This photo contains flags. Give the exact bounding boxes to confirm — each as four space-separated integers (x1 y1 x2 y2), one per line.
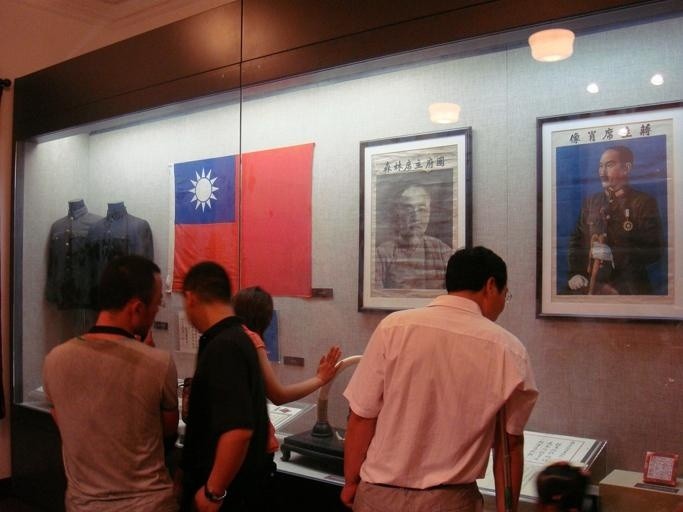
165 140 314 303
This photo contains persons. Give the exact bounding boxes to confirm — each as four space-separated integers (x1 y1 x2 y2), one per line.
233 284 342 511
173 261 272 511
42 254 181 510
42 200 154 311
534 462 592 512
340 246 540 511
375 184 452 288
565 145 664 294
132 260 163 348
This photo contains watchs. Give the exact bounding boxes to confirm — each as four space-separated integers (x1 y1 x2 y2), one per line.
203 483 228 503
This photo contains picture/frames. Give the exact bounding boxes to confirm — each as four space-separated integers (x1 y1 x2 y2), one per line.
358 125 473 313
536 108 682 323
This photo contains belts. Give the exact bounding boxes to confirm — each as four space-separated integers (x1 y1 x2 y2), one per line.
376 481 477 492
